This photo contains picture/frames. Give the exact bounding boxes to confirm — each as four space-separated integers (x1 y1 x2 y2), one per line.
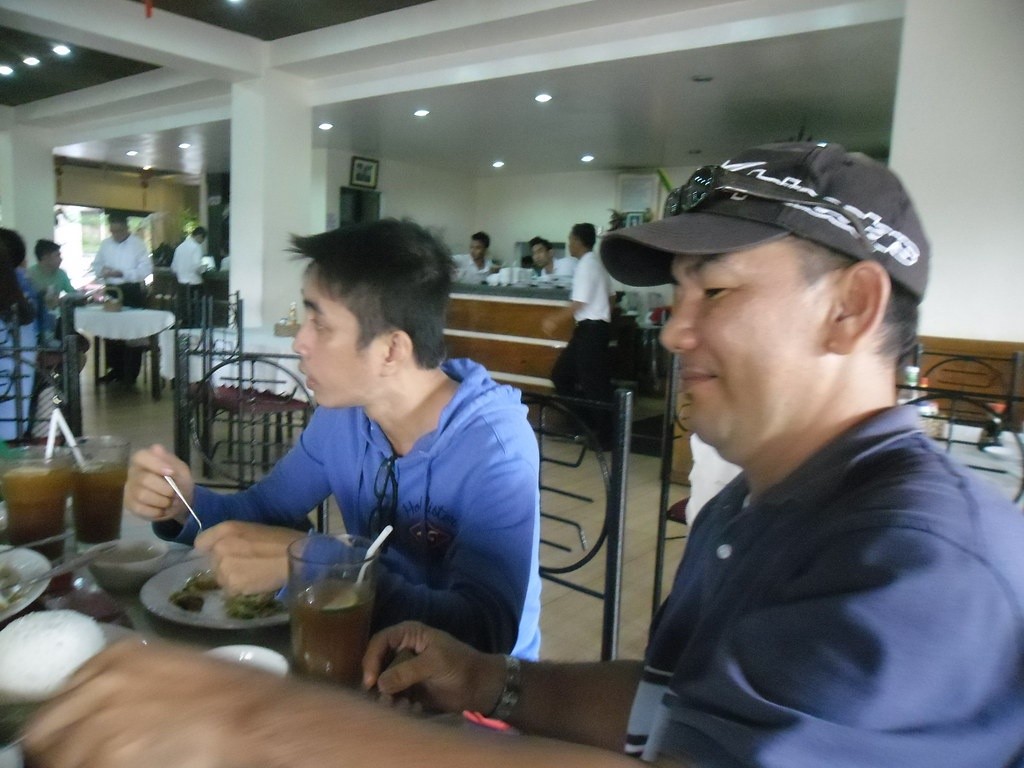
625 212 643 227
348 156 380 189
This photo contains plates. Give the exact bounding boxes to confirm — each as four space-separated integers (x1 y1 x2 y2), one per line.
71 542 190 596
136 553 289 630
0 543 52 622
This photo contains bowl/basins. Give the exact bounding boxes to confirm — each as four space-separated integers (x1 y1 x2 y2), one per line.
201 644 291 679
487 266 535 287
82 538 168 592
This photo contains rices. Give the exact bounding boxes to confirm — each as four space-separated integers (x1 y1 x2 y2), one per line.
0 608 106 708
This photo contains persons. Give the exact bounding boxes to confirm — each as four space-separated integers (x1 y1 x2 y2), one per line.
527 236 578 277
121 216 541 662
170 226 208 328
446 231 493 283
0 227 91 375
19 140 1024 768
92 212 154 384
549 223 617 429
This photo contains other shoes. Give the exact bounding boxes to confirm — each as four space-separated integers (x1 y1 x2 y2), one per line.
98 370 137 386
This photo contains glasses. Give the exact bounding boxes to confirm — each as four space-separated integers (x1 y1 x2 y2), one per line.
367 454 406 553
662 164 872 255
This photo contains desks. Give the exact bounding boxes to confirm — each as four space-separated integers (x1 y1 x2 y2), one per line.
686 422 1024 526
74 302 176 402
160 327 313 408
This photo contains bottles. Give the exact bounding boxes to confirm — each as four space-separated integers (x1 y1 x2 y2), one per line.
977 403 1004 450
893 366 920 407
288 302 297 329
918 377 929 400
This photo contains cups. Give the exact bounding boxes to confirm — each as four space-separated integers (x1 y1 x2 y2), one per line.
63 434 131 542
287 533 381 688
0 445 73 569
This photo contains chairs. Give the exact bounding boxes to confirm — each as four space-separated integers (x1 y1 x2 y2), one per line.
0 284 1024 663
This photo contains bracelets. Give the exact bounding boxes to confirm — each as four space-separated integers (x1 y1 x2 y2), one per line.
488 653 522 720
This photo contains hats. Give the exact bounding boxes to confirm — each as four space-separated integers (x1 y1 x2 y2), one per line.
599 141 932 302
